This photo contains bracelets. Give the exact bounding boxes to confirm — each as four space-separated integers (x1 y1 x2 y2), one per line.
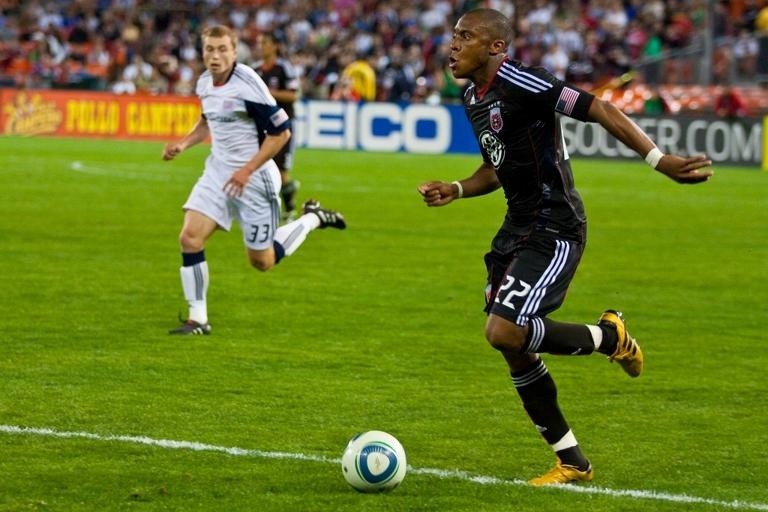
451 177 465 199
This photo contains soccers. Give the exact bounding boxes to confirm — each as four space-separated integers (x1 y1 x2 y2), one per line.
341 428 406 492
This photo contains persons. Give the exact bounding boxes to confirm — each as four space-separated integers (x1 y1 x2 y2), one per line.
162 25 347 335
0 0 768 116
416 9 715 487
247 29 301 225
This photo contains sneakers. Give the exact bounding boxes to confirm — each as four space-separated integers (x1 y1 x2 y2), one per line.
304 200 345 229
597 309 643 377
529 457 592 486
285 209 296 223
171 320 210 335
